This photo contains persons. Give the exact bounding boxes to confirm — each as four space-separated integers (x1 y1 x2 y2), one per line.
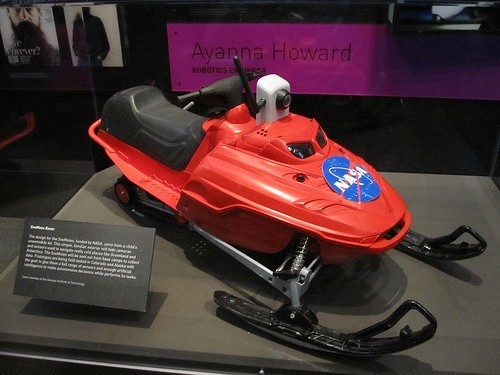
6 4 61 66
72 5 110 67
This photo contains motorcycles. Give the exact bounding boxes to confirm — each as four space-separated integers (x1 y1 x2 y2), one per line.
88 70 489 362
